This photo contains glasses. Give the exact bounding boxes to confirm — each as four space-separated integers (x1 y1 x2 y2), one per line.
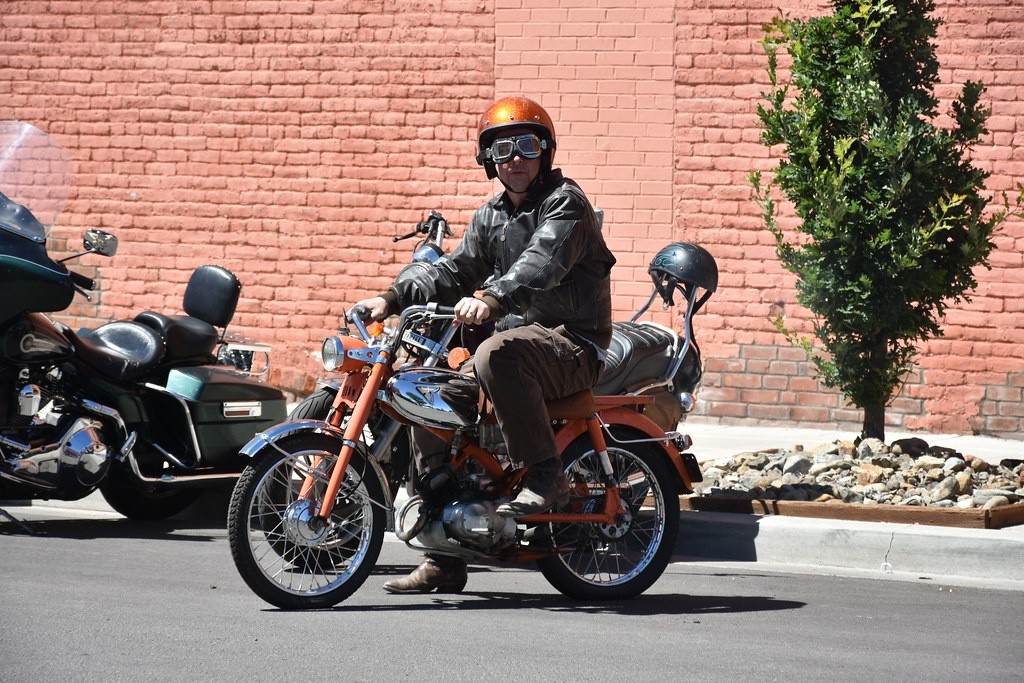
476 133 557 165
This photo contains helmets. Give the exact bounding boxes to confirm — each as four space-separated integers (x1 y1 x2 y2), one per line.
476 96 556 180
648 242 718 293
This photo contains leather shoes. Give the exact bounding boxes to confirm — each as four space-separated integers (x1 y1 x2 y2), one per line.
495 455 571 516
382 556 468 593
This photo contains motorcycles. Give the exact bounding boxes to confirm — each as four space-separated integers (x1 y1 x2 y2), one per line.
230 212 719 614
0 120 287 537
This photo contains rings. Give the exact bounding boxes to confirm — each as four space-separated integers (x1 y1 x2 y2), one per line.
468 312 475 315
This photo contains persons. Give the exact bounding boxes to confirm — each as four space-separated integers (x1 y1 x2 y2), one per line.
347 95 617 595
